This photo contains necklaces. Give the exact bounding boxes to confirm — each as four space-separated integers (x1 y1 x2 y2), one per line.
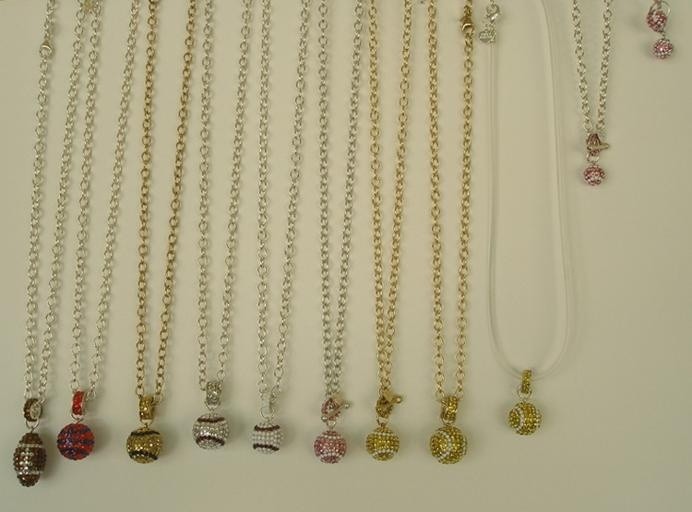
126 1 196 463
314 0 363 462
427 0 474 462
57 2 137 460
572 0 612 184
488 0 569 435
367 1 413 462
250 1 310 453
13 3 86 488
192 0 253 449
